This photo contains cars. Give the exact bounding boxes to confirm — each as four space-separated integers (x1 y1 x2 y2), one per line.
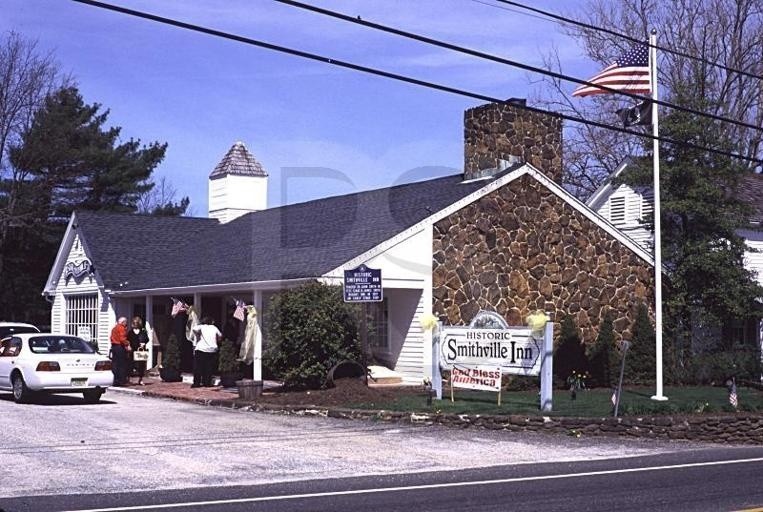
0 332 116 403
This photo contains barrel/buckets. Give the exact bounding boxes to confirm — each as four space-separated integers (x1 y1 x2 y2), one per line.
235 380 263 400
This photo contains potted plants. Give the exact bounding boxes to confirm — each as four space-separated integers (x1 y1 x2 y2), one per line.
159 332 183 382
217 338 240 387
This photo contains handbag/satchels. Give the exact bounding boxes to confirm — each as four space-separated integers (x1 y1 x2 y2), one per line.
133 347 150 362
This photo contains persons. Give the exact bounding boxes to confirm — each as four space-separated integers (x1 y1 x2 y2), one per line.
189 317 223 388
110 316 150 387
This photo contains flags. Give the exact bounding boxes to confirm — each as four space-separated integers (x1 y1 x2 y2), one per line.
170 296 189 319
232 300 246 322
728 382 738 409
570 40 652 98
610 389 617 407
